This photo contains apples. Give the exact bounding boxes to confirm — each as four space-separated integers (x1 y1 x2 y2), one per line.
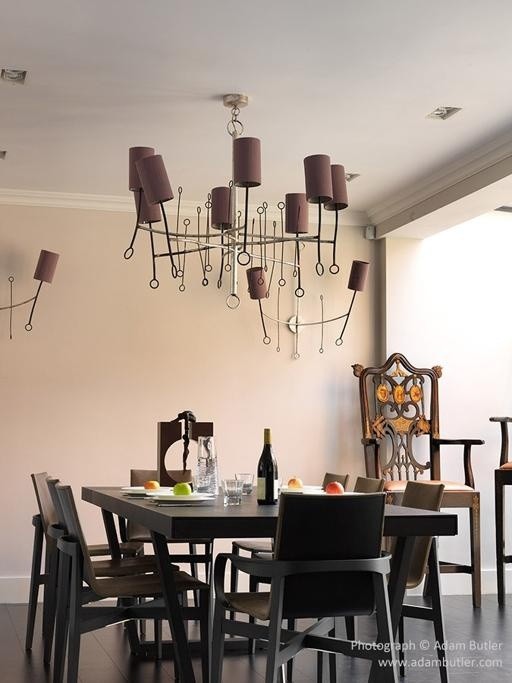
326 482 344 494
174 483 192 495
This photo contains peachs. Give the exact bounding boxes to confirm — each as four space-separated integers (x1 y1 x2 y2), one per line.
144 480 159 489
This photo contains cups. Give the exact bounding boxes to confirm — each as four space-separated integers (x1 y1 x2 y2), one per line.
235 474 254 496
192 475 212 493
197 436 220 497
221 479 244 507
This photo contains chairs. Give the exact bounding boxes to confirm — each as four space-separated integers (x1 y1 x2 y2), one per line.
487 410 512 616
22 466 459 682
352 350 487 619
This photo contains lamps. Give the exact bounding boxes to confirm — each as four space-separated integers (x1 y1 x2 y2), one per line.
115 89 354 310
242 253 371 357
1 246 60 343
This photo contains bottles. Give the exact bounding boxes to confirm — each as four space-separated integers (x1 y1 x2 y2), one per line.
256 427 279 506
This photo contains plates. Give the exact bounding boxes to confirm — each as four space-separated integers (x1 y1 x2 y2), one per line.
282 485 363 495
119 486 172 495
141 492 216 504
